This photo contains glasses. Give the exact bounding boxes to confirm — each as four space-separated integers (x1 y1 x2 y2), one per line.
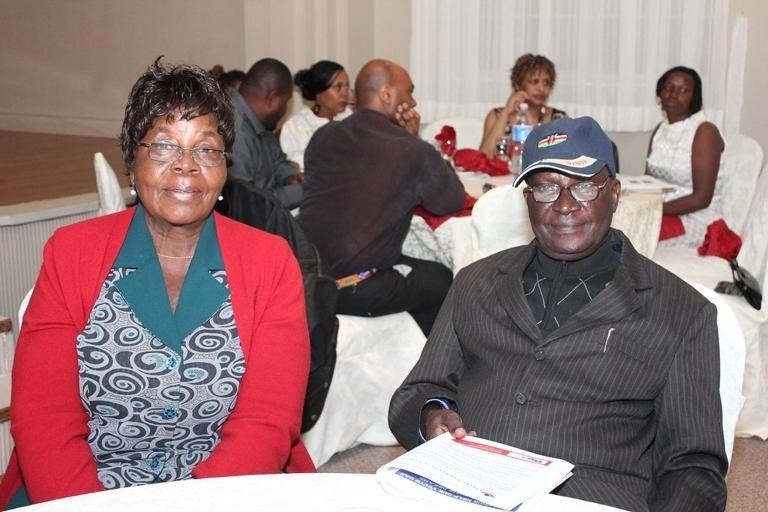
529 182 604 203
141 144 228 167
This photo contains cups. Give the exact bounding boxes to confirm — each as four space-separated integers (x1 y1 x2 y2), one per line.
439 139 457 162
495 136 510 156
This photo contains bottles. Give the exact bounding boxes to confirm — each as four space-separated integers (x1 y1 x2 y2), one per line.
511 104 531 176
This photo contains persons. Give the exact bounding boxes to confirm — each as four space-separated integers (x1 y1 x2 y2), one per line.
642 65 725 248
387 116 728 511
211 58 357 247
479 50 566 174
292 57 464 337
1 56 320 512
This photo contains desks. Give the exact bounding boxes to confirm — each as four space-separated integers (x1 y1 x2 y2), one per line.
444 159 748 483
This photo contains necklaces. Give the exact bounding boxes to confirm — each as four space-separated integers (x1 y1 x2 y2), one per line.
158 253 193 260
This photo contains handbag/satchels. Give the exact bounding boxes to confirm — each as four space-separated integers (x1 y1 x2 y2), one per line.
730 259 761 309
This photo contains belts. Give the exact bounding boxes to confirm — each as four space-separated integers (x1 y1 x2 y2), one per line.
334 268 379 290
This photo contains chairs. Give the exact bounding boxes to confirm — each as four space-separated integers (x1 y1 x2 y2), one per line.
665 127 766 441
92 149 439 474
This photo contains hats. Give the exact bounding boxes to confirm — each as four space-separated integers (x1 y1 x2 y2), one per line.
512 116 616 188
